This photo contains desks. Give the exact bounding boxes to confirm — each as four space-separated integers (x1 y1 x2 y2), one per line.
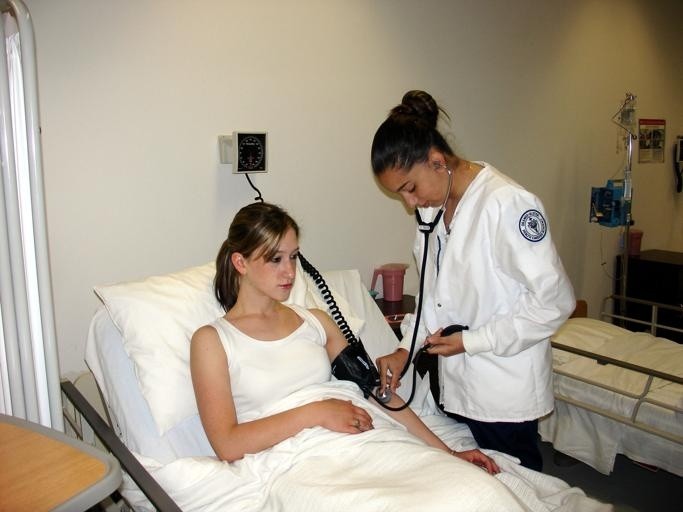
0 413 123 512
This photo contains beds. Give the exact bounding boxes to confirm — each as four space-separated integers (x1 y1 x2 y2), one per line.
537 294 683 478
59 269 612 512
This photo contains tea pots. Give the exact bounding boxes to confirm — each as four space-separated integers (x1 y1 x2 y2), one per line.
371 263 409 302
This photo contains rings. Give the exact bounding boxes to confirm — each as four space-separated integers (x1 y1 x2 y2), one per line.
354 418 360 427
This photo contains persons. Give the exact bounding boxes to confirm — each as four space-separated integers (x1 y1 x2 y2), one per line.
371 88 579 470
187 199 502 476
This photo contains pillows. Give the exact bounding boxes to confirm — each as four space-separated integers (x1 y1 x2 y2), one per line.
94 258 366 437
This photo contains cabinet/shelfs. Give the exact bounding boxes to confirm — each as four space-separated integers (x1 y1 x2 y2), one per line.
614 249 683 344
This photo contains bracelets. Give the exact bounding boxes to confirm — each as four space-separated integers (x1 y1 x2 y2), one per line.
450 450 457 456
